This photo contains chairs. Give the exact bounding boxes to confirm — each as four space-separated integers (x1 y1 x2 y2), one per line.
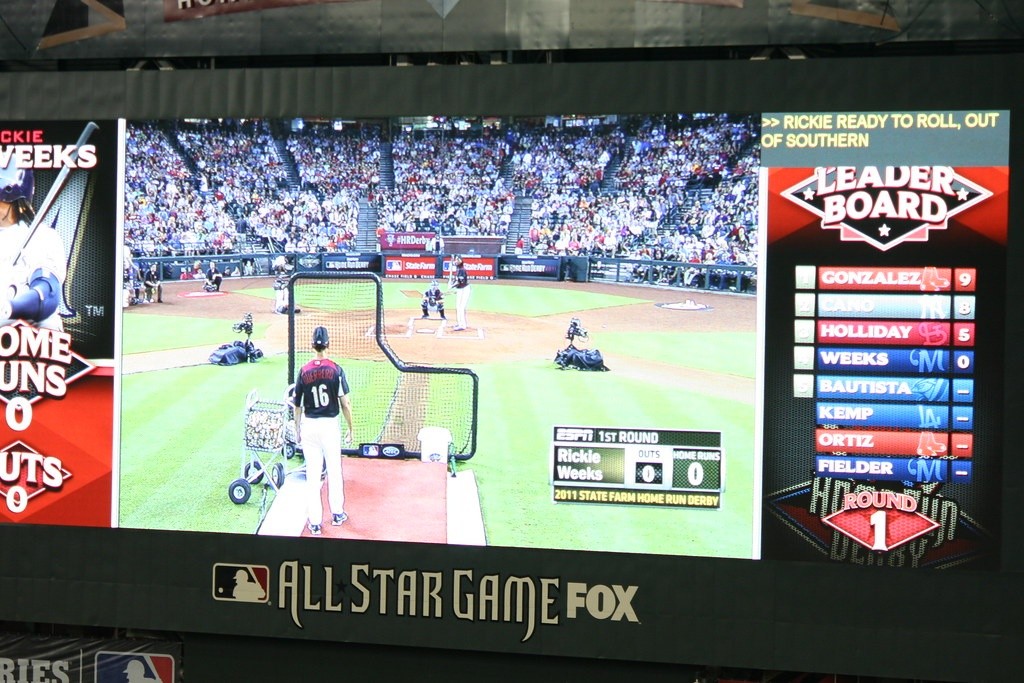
121 121 759 300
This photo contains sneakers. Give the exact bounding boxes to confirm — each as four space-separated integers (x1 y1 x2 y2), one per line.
332 513 347 526
308 523 321 535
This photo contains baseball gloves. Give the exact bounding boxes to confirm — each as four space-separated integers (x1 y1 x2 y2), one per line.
429 298 436 306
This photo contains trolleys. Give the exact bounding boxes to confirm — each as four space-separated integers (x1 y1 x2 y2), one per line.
227 386 291 504
279 383 305 460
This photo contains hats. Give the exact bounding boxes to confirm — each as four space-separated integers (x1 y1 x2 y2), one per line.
312 326 329 345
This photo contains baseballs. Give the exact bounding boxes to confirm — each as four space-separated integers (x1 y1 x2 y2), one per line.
246 411 286 448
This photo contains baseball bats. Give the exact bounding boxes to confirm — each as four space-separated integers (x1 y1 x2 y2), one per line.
10 122 98 269
448 253 455 290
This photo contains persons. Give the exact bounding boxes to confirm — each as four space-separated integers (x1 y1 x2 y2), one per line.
205 262 222 292
450 255 470 331
420 281 447 321
125 125 758 290
2 154 66 326
143 264 163 303
291 327 355 536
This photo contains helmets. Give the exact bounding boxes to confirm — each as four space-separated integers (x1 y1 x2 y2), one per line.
430 281 439 292
454 255 463 266
1 152 36 204
